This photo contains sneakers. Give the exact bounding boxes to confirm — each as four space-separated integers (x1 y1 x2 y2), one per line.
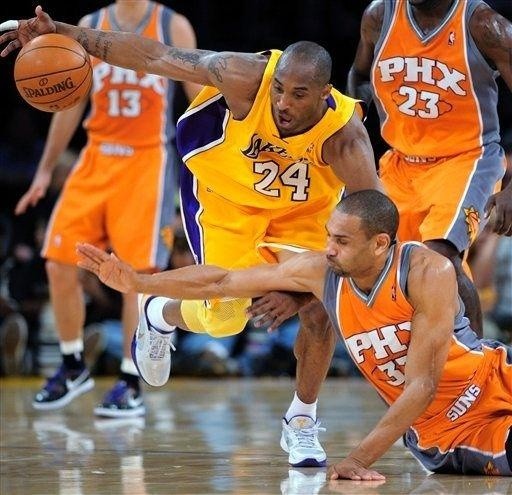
94 379 147 417
130 293 177 390
280 412 330 467
31 367 94 411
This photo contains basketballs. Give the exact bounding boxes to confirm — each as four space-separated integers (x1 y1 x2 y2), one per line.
14 33 94 112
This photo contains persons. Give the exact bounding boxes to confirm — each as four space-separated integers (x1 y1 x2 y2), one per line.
75 189 512 482
0 6 381 466
14 0 207 418
342 1 512 341
